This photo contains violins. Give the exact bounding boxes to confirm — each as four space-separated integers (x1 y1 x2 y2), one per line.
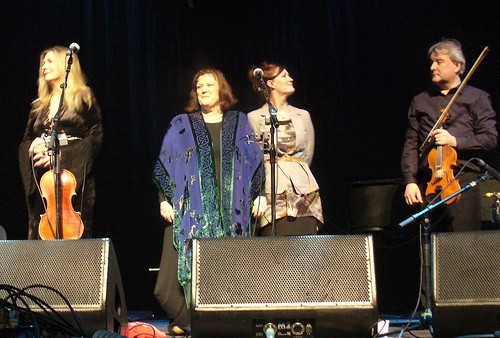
37 118 85 240
425 109 462 207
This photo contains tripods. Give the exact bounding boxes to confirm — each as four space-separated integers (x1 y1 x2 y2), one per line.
380 169 493 337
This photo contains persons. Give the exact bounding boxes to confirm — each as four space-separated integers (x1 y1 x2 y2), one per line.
17 46 102 240
247 64 324 237
401 41 499 311
153 67 267 336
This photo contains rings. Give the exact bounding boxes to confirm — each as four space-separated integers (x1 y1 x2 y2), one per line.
42 151 46 158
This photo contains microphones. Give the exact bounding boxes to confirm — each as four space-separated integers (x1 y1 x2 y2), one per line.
476 157 500 183
69 42 80 53
252 67 266 90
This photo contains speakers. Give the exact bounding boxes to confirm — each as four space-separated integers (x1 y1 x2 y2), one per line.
428 230 500 338
0 238 129 338
189 234 378 338
